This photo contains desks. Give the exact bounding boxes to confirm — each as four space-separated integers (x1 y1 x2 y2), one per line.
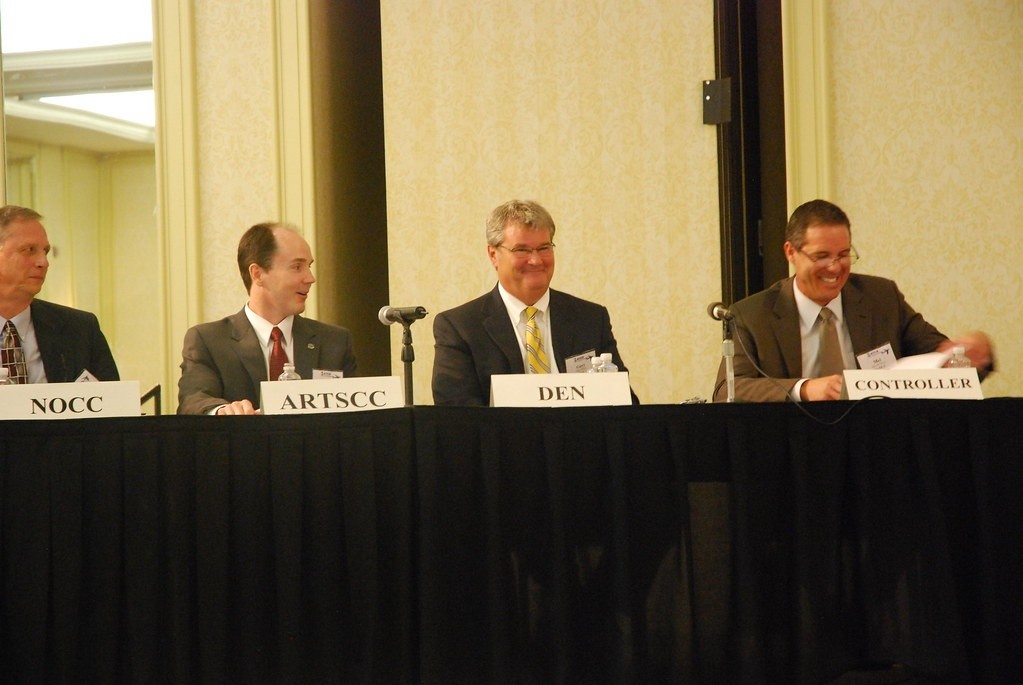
1 396 1022 685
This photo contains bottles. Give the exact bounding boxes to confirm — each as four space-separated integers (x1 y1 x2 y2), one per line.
599 351 618 372
584 356 608 372
277 362 301 380
947 346 971 368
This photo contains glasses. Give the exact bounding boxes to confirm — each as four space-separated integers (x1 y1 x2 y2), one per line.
799 245 858 267
499 242 555 257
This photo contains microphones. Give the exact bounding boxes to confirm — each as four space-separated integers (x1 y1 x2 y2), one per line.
707 301 734 322
378 305 429 327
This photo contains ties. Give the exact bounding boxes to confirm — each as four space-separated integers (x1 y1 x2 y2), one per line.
522 306 551 373
811 308 849 400
1 320 30 384
270 326 288 381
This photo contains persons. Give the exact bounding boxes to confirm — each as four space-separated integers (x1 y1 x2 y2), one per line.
711 200 992 402
176 222 363 415
0 205 120 384
431 200 640 407
